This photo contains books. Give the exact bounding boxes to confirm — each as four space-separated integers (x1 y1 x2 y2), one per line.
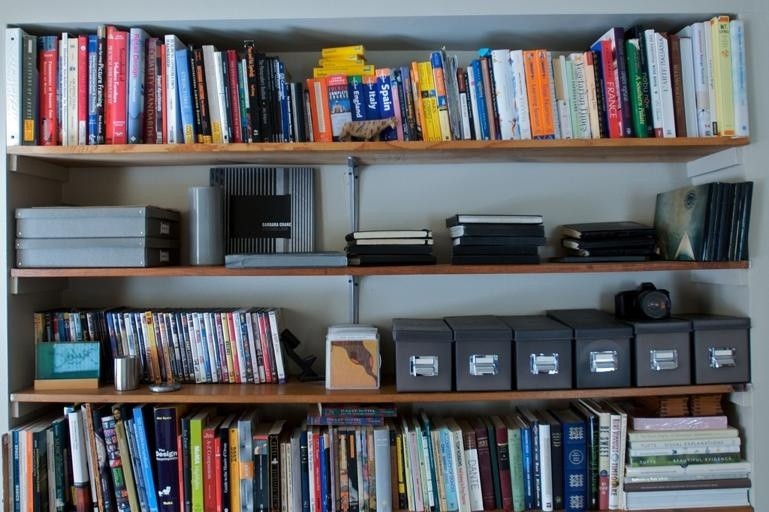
653 181 753 262
446 213 546 266
344 229 437 267
549 222 657 263
31 306 288 386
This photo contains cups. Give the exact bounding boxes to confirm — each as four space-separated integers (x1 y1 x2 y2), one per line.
113 354 140 392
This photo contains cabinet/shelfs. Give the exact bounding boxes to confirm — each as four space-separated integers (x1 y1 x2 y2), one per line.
0 1 769 511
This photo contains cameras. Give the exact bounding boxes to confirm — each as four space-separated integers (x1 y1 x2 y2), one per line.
614 283 671 323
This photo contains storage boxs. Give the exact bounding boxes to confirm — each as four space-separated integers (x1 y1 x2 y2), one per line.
675 311 750 385
392 318 453 393
326 327 382 390
444 315 513 392
616 311 692 388
547 308 631 389
15 239 180 268
501 314 575 390
14 206 181 238
